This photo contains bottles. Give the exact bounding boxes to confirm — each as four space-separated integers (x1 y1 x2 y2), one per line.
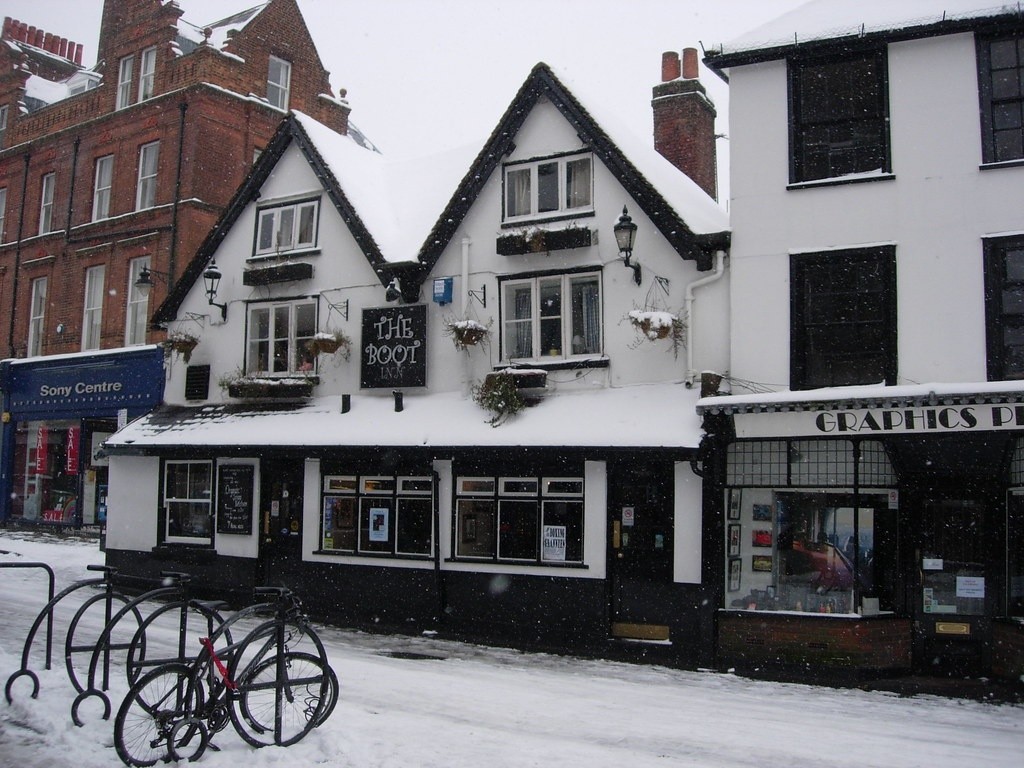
796 602 802 611
826 604 830 613
820 603 824 612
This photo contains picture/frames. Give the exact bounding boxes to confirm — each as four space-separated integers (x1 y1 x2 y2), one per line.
727 487 742 520
727 557 741 593
727 523 741 557
752 555 772 572
462 514 477 544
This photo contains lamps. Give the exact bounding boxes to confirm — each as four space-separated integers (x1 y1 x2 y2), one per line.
202 257 228 322
135 263 172 297
613 203 642 288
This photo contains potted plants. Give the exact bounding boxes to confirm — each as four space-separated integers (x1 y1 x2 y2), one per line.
436 297 495 360
305 323 354 365
219 365 314 398
162 332 199 366
617 279 691 361
471 372 546 429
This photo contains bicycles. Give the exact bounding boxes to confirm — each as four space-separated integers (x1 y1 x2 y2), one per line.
113 587 340 767
